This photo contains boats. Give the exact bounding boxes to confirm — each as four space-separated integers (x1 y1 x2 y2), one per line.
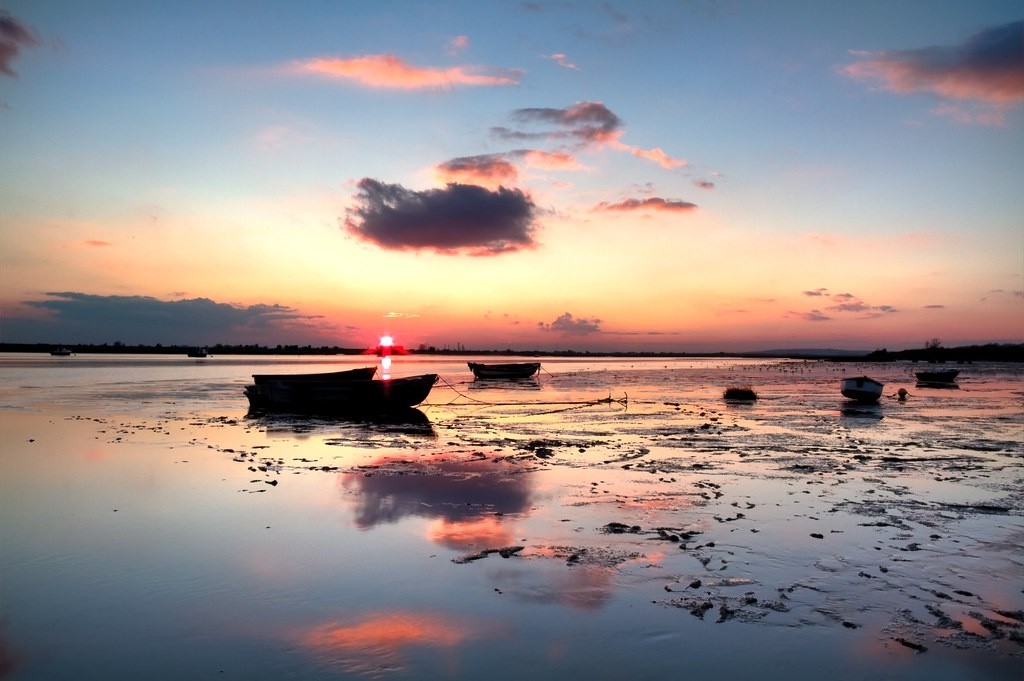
465 360 541 380
839 375 885 401
251 366 378 389
914 368 963 382
242 373 438 415
